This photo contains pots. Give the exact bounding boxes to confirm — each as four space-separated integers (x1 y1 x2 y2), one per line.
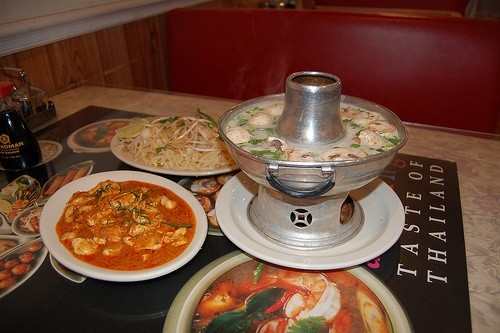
216 71 410 250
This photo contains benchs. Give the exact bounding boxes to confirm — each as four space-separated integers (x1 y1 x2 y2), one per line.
167 0 500 135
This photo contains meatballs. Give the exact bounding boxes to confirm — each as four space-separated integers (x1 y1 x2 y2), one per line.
0 242 41 290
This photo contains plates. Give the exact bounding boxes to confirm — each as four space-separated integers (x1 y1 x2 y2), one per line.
215 172 404 270
110 116 241 177
38 170 209 282
0 160 93 298
177 170 240 236
162 248 414 333
0 140 63 171
67 118 131 153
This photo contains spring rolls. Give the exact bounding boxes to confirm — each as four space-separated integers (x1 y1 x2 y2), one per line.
44 167 90 194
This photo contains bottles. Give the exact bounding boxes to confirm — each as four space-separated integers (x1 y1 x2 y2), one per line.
0 82 49 188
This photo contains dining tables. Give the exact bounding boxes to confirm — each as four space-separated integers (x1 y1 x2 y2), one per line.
0 80 500 333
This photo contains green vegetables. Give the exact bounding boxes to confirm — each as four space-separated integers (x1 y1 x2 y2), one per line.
197 264 327 333
0 177 41 209
156 109 224 151
95 183 193 227
